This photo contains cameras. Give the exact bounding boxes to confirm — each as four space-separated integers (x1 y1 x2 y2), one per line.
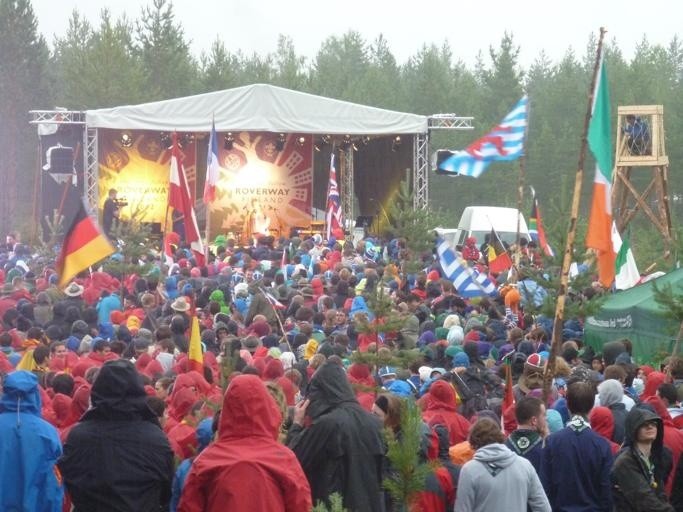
113 197 128 208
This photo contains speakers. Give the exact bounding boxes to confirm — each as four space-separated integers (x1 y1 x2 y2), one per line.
48 148 73 175
431 151 457 175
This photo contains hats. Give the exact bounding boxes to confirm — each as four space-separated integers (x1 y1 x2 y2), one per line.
379 365 397 376
65 282 84 297
277 285 290 299
525 355 547 370
171 297 190 311
302 289 315 297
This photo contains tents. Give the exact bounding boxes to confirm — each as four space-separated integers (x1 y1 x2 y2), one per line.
29 83 475 244
581 267 683 370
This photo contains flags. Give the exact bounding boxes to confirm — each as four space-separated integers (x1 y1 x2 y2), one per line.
326 151 346 241
480 226 532 292
187 296 205 369
170 135 206 268
435 90 530 181
257 285 290 311
540 26 641 290
54 194 121 290
527 188 558 260
202 123 223 252
434 231 507 318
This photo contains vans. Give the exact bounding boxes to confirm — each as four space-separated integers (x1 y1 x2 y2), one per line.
454 205 532 259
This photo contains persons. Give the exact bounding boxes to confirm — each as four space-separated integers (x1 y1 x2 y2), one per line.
506 396 547 469
1 233 51 391
471 274 510 444
644 397 683 502
142 235 222 465
423 381 471 449
291 355 387 510
51 284 180 430
399 412 461 511
179 375 312 511
612 406 671 512
63 360 174 511
539 382 615 512
456 419 551 512
498 288 683 444
375 231 484 413
2 370 63 510
206 231 379 415
102 187 118 234
370 393 405 436
622 116 651 158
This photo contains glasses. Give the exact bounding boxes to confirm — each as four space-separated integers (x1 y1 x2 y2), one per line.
641 420 659 427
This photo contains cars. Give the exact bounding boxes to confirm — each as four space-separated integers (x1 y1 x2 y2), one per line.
529 230 555 257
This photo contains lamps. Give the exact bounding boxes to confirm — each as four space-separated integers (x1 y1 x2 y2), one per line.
274 133 286 150
177 132 195 149
352 136 370 152
391 135 401 153
158 131 174 152
224 132 234 150
120 131 133 148
338 135 351 153
296 134 305 147
314 134 330 153
